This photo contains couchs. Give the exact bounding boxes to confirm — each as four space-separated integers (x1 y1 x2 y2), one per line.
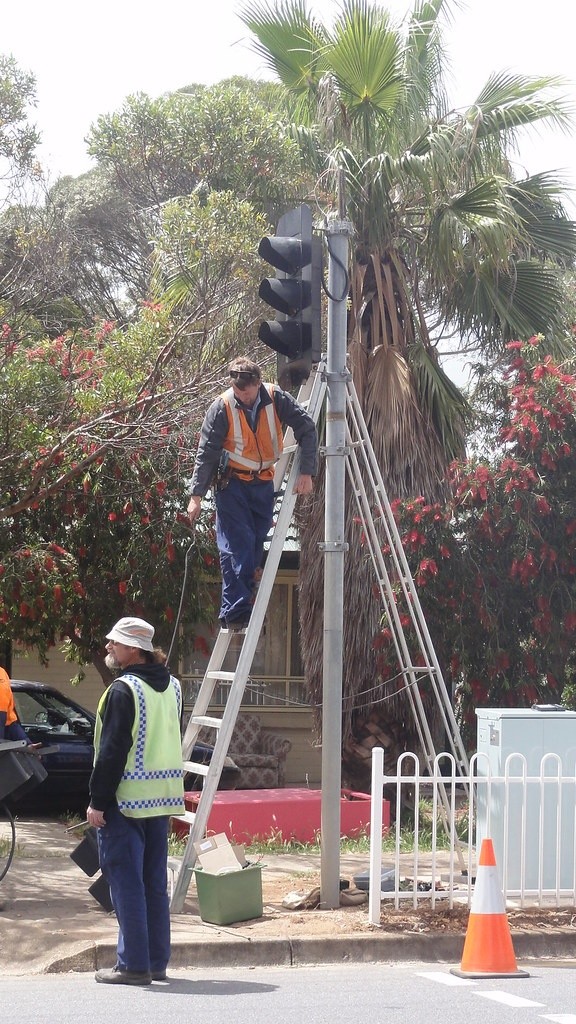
190 714 293 788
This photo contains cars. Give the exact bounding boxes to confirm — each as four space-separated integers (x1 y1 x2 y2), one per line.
10 678 242 812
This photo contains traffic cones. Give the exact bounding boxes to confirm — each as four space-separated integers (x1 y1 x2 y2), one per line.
449 839 531 979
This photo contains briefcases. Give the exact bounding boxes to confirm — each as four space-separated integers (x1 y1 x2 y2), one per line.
353 867 401 891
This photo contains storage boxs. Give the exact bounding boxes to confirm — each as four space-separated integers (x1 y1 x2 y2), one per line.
354 868 395 892
188 860 267 925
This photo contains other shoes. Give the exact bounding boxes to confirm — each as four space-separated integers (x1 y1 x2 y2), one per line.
220 611 268 629
96 964 151 985
151 969 167 979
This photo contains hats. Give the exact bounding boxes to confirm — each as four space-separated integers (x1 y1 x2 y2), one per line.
106 615 155 652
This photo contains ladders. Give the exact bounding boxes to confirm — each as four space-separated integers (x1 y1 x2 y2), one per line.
169 356 475 917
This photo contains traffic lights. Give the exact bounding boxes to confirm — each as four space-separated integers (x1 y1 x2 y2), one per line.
255 205 323 381
0 740 49 806
69 825 114 913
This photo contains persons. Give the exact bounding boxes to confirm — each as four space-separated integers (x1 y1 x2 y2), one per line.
84 614 188 987
1 664 43 762
186 354 320 631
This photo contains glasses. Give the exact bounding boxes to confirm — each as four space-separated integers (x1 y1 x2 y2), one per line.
110 639 120 646
230 370 260 380
